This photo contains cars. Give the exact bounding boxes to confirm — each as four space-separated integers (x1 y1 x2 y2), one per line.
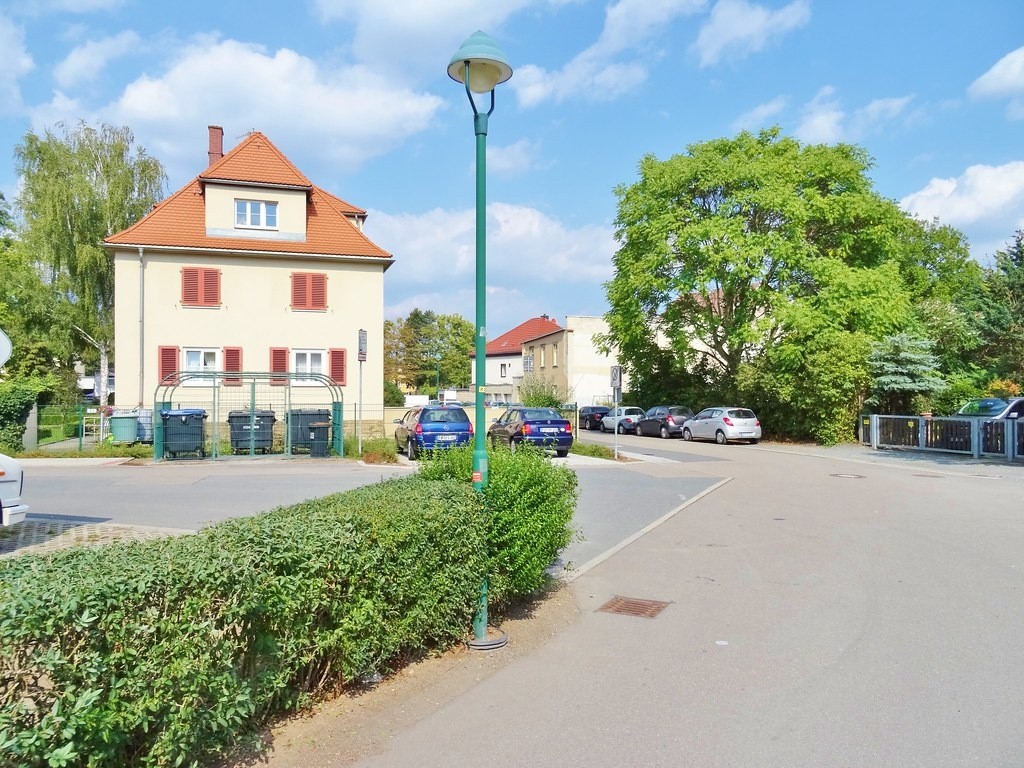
431 399 576 410
634 404 696 440
392 404 475 461
681 406 762 445
939 396 1024 450
599 406 646 434
578 405 612 431
486 405 574 457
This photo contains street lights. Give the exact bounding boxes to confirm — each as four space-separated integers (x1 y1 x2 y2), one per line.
434 353 442 405
445 28 515 640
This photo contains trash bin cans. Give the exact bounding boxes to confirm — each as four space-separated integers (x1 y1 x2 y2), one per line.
159 409 207 461
285 408 332 458
110 416 138 446
227 409 278 455
